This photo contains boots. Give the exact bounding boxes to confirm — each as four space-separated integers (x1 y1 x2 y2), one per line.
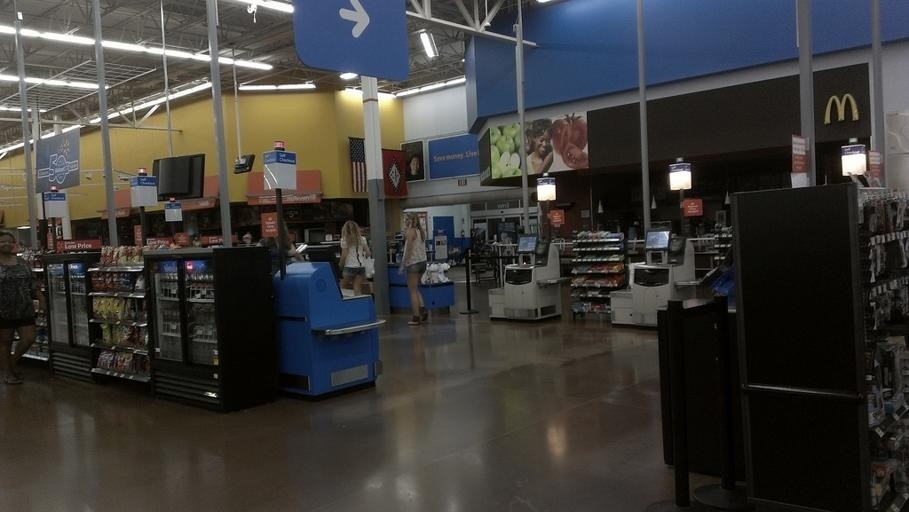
407 304 429 325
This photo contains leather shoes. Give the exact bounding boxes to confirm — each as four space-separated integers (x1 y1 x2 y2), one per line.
2 372 25 385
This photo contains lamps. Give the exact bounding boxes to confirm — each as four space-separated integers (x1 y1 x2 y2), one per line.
418 27 439 64
2 25 272 158
393 74 466 103
336 70 359 86
245 0 295 20
236 83 315 93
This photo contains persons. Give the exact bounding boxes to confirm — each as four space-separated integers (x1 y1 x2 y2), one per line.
523 119 553 174
338 220 371 296
405 154 422 179
396 211 430 327
1 231 50 386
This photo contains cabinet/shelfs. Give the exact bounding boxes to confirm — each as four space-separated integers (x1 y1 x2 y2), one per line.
573 230 630 319
19 240 276 411
661 188 908 510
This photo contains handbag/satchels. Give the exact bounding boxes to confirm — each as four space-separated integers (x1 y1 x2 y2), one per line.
362 252 376 280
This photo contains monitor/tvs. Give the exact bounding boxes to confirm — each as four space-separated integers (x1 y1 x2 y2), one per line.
305 244 344 281
517 234 540 254
643 228 671 251
152 153 205 202
234 154 255 175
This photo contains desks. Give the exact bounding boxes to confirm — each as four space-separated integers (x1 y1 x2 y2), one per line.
470 224 651 290
376 229 451 272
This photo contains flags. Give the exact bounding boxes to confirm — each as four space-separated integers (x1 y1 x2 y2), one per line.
349 137 367 194
381 149 409 197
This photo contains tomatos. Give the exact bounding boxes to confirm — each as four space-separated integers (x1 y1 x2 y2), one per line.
562 143 589 169
552 113 588 153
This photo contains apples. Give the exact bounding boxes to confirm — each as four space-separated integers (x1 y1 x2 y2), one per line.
489 123 522 178
